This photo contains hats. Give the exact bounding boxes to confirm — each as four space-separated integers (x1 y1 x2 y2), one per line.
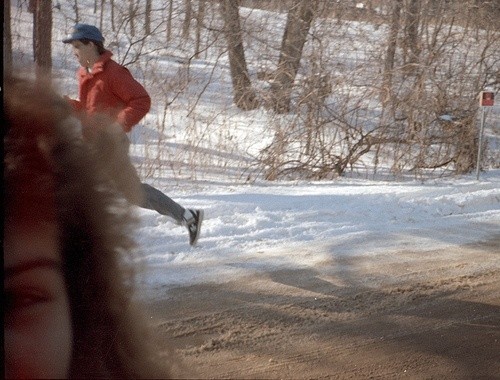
60 23 106 44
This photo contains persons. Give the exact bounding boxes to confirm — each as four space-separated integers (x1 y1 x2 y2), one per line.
60 22 205 245
2 73 172 380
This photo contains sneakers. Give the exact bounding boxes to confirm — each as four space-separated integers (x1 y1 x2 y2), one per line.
185 209 204 246
